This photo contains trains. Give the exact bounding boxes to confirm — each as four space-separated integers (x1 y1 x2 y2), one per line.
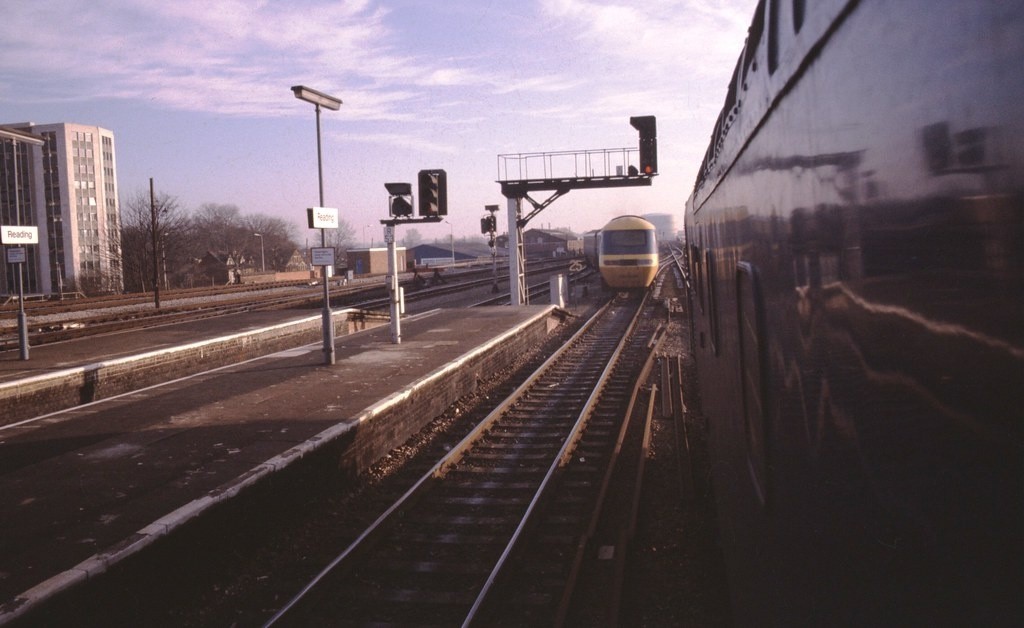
584 214 659 298
686 0 1023 627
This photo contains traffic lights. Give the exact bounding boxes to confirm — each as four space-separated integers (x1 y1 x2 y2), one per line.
630 115 657 175
421 173 438 219
481 216 497 234
